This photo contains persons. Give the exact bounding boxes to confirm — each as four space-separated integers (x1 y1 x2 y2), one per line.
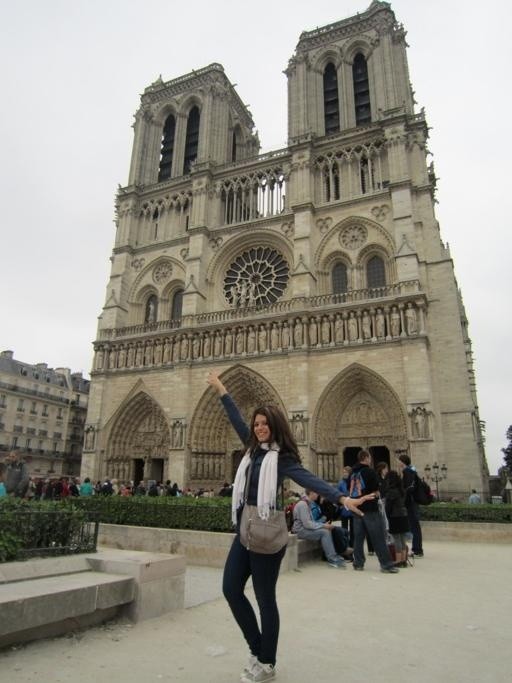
1 461 9 499
467 487 483 504
203 366 377 683
24 475 232 502
1 448 33 495
279 446 436 573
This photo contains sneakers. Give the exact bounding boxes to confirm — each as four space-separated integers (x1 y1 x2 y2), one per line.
410 551 424 558
327 555 347 569
239 653 278 682
381 566 399 573
339 546 354 556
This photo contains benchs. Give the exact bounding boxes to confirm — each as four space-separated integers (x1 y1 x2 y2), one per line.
298 534 322 563
0 571 134 647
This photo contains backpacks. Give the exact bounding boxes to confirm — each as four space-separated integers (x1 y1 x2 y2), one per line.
410 469 433 505
283 496 311 533
348 468 367 499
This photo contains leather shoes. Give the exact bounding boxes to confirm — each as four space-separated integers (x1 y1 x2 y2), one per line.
393 560 407 567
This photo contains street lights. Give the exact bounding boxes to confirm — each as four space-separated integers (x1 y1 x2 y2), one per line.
424 462 447 502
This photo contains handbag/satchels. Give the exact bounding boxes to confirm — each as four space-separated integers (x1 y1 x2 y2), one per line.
238 501 289 554
